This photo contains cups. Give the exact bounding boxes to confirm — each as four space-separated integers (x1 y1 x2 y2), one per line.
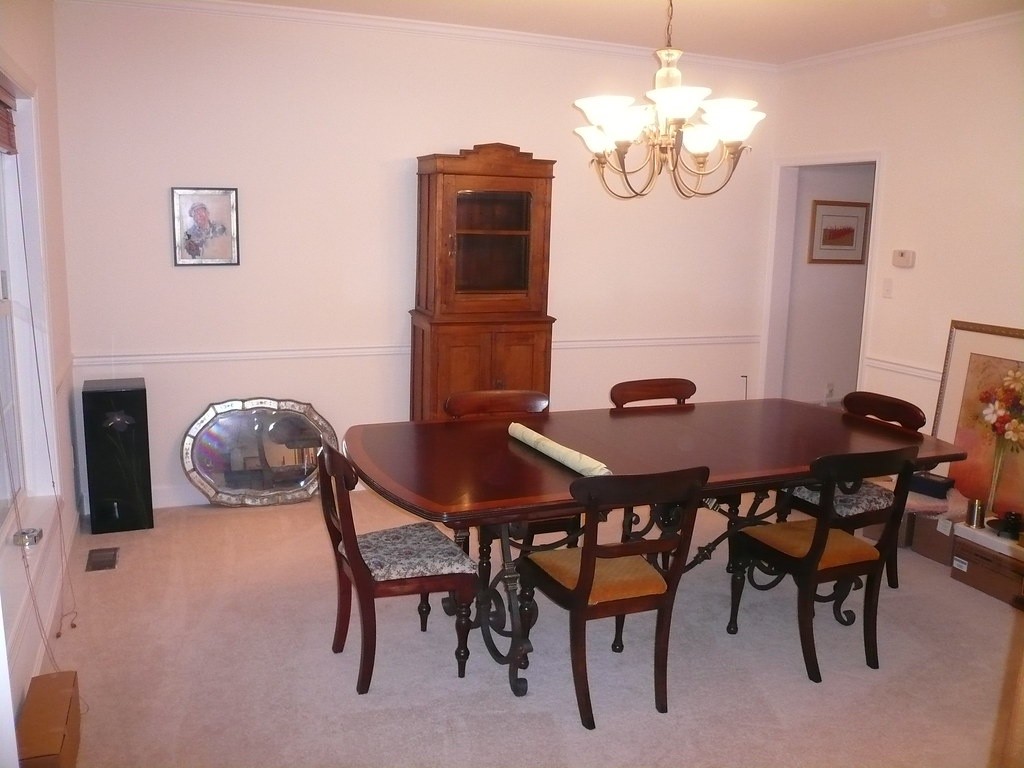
965 498 986 529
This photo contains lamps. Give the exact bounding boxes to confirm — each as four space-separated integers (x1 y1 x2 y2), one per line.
572 0 766 205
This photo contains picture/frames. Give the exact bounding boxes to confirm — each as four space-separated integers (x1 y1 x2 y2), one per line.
213 452 232 473
169 186 244 270
243 455 262 470
807 199 870 269
921 320 1024 518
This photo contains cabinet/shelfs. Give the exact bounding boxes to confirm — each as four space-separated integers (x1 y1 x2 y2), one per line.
402 142 564 424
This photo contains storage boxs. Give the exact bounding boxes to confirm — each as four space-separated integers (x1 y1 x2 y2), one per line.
862 506 913 550
949 537 1024 613
15 669 84 768
81 378 155 536
953 514 1024 563
911 510 998 568
866 475 892 483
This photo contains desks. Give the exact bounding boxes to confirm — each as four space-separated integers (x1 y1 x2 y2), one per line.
343 396 966 669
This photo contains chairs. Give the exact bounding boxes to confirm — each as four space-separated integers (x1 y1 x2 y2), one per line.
726 444 920 687
773 391 927 594
253 414 323 492
439 391 584 612
608 376 745 581
514 468 711 734
315 438 481 700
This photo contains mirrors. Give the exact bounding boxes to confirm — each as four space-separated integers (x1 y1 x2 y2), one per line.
183 398 338 508
